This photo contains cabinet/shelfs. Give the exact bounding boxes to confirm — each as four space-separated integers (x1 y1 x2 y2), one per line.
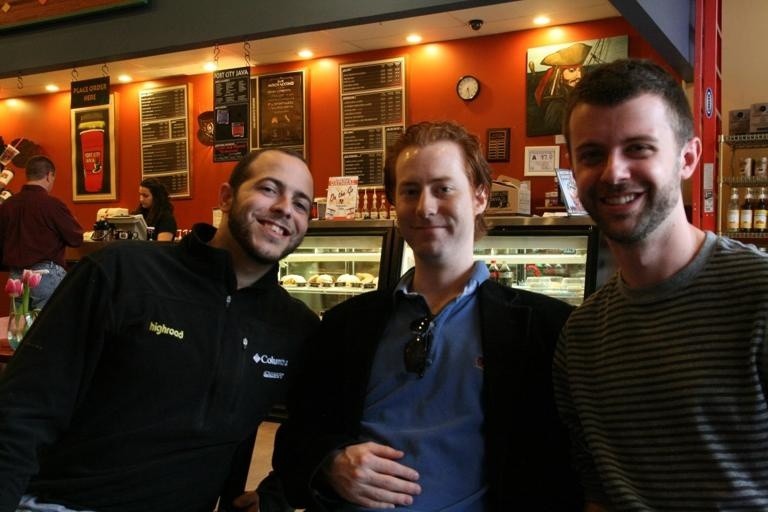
723 134 768 238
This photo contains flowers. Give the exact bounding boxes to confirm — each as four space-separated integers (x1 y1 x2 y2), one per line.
6 269 42 313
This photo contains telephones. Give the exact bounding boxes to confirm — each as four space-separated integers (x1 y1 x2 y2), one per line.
94 221 111 230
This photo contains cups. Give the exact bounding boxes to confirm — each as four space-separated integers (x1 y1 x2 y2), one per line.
146 226 155 241
216 110 230 125
317 199 327 221
0 144 19 166
231 123 244 138
79 129 105 192
0 190 13 204
0 167 15 188
175 229 192 241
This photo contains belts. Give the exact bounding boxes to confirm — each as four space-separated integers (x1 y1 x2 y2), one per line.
38 259 56 265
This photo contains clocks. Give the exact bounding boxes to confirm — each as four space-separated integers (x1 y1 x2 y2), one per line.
457 75 480 101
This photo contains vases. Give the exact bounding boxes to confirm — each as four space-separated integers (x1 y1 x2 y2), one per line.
7 313 38 352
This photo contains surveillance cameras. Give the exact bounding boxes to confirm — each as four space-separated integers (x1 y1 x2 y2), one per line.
469 20 482 30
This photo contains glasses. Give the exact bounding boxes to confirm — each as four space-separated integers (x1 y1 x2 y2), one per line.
401 314 435 377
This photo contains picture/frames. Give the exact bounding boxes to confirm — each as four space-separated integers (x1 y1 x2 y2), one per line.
524 146 560 176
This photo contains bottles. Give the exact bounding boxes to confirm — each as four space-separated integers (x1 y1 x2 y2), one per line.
499 261 512 287
543 263 562 276
355 187 396 221
489 259 498 284
725 187 767 232
544 190 557 207
100 215 105 221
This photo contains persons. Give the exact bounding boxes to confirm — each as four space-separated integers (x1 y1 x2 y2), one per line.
548 56 768 512
0 145 321 512
271 117 578 512
0 155 84 311
131 176 177 241
